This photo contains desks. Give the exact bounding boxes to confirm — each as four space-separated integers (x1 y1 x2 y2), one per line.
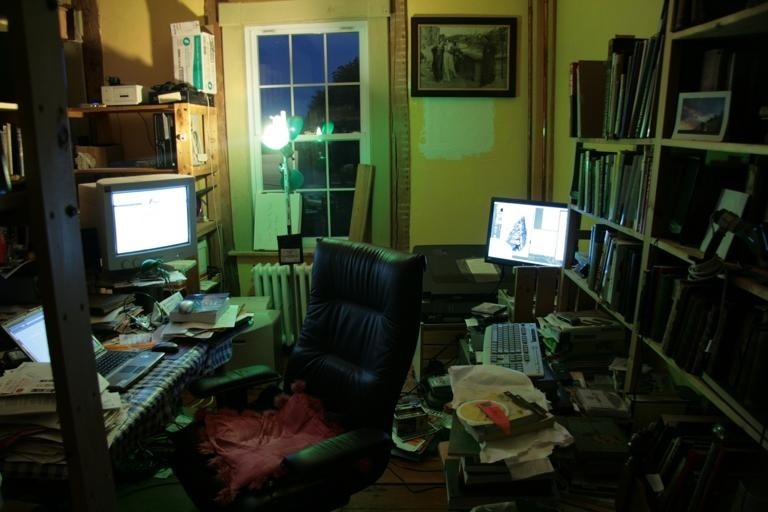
1 294 280 482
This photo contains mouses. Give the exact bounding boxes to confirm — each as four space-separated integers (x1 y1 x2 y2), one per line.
178 299 195 314
151 341 180 355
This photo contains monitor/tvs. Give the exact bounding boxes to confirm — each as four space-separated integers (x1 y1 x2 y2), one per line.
78 173 198 273
483 196 568 269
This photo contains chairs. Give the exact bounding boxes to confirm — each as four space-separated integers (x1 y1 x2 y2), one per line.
164 236 426 512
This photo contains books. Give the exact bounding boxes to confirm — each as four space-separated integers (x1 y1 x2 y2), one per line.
88 292 137 317
1 122 26 191
170 293 230 324
570 143 652 236
638 262 719 373
573 221 643 322
569 31 663 139
718 307 767 431
437 363 554 511
633 408 764 511
574 387 628 417
555 417 632 511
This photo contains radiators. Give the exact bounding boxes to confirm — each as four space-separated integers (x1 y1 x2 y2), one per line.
293 262 314 340
250 262 295 347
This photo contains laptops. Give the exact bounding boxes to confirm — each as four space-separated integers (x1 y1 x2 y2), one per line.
0 305 165 392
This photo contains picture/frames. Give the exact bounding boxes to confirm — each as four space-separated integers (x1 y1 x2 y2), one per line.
410 16 516 98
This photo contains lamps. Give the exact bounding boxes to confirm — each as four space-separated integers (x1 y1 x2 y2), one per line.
260 110 305 263
315 121 335 160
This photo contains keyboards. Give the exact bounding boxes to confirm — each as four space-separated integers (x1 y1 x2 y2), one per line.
482 322 545 377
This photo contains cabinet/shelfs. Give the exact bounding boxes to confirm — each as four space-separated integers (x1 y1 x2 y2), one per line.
510 0 768 454
0 0 241 298
